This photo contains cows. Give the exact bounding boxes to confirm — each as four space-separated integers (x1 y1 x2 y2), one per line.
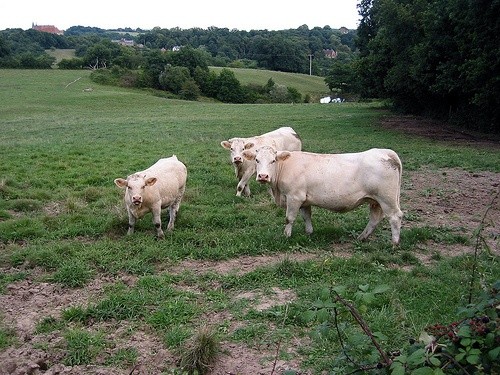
241 144 403 245
113 155 187 240
220 126 302 196
